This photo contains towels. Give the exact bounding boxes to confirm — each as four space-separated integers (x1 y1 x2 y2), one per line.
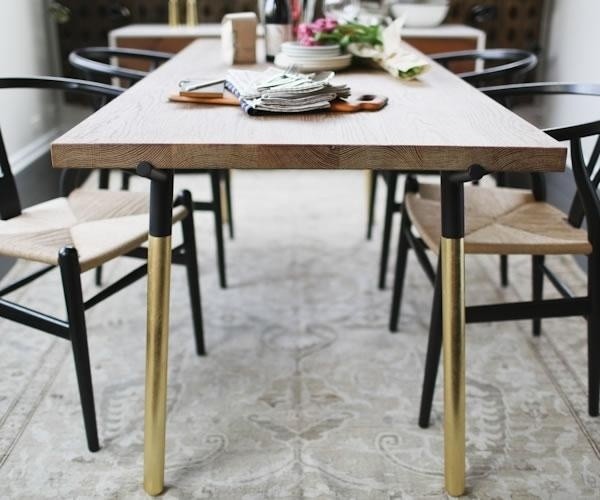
222 67 351 115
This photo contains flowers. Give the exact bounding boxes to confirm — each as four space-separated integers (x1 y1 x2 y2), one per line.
299 16 431 81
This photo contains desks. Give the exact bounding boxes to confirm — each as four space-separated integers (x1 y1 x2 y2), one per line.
106 22 489 87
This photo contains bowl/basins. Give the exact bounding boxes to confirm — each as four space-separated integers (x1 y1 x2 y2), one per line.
391 4 450 28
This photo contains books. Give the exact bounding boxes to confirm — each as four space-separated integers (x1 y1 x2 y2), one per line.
180 71 351 116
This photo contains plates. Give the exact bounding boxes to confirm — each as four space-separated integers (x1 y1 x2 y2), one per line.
273 42 352 73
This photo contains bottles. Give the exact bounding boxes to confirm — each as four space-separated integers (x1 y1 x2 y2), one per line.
264 0 290 62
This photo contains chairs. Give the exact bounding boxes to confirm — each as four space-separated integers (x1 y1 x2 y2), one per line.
390 82 600 428
68 46 234 288
0 75 205 454
367 48 539 290
